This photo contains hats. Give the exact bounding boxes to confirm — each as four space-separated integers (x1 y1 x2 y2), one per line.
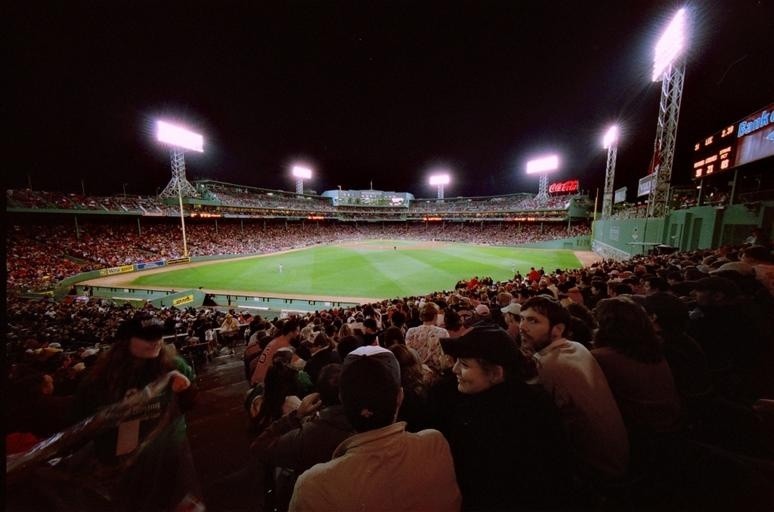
476 303 490 316
131 311 164 340
682 261 756 292
300 331 328 347
501 302 521 315
341 344 402 394
441 322 523 362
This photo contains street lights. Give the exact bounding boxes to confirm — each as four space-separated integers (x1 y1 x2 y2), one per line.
429 174 450 203
154 119 205 199
291 165 312 198
645 7 687 219
526 154 560 202
599 124 620 218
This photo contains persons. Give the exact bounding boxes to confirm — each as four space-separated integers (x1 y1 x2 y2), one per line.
0 181 743 292
1 230 773 512
